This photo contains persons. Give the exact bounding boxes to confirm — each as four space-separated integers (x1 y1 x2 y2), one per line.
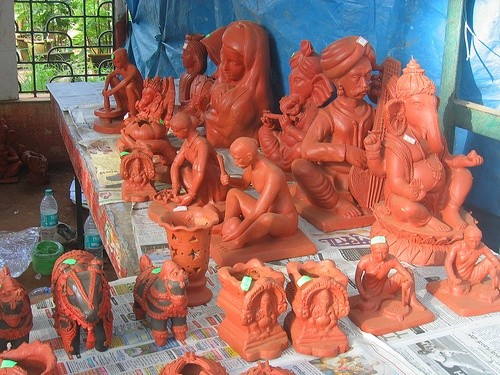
178 33 212 117
290 36 383 220
219 137 298 252
103 48 143 118
355 236 424 310
445 223 500 291
260 40 333 173
168 113 228 206
204 20 271 148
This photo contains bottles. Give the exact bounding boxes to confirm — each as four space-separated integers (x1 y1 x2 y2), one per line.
40 189 58 241
83 210 104 269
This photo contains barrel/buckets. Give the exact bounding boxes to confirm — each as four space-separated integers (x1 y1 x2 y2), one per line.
32 240 64 276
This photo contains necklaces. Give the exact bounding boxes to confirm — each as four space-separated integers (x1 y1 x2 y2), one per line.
374 261 384 269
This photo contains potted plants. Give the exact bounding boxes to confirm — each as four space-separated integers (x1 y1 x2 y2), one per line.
14 0 112 70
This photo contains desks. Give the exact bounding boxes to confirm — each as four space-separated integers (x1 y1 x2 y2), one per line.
30 79 500 375
